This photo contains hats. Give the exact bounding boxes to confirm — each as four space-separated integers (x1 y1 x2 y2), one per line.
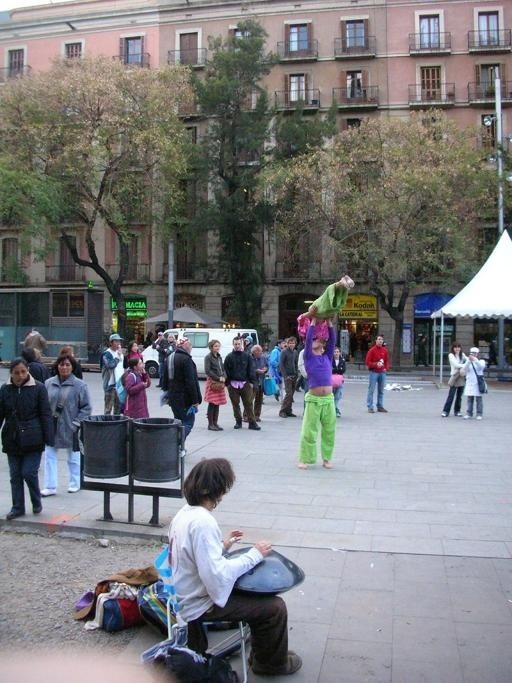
470 347 480 353
109 334 124 342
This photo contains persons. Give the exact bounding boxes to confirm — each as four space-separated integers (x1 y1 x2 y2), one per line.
163 337 202 457
167 458 303 676
350 332 368 362
40 356 92 497
460 346 486 420
0 356 55 518
297 316 337 470
365 336 389 414
441 342 469 417
205 336 346 431
21 328 83 382
99 328 175 417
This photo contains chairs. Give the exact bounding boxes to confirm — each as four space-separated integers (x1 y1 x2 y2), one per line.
154 543 251 683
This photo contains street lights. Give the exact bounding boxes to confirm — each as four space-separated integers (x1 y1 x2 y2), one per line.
482 76 511 372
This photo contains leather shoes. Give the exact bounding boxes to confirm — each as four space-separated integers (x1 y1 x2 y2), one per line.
287 414 296 417
234 421 242 429
249 422 261 430
216 425 223 430
208 425 218 430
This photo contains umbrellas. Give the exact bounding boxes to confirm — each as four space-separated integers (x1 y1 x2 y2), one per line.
138 305 230 325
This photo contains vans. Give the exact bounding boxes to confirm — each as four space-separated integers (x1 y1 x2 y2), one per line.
138 325 260 380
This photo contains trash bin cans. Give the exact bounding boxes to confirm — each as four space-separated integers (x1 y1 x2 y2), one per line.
133 417 182 482
80 414 130 478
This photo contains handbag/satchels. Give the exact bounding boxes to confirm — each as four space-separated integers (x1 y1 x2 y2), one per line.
100 596 145 633
263 372 275 396
209 376 226 392
477 375 488 393
164 647 240 683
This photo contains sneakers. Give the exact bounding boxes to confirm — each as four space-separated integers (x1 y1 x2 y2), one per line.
40 488 56 496
33 505 42 514
368 409 375 413
377 407 387 412
249 649 295 664
69 488 79 493
6 511 24 519
251 656 302 676
441 413 483 420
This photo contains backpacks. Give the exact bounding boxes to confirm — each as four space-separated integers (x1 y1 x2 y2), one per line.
115 373 136 404
137 582 182 638
